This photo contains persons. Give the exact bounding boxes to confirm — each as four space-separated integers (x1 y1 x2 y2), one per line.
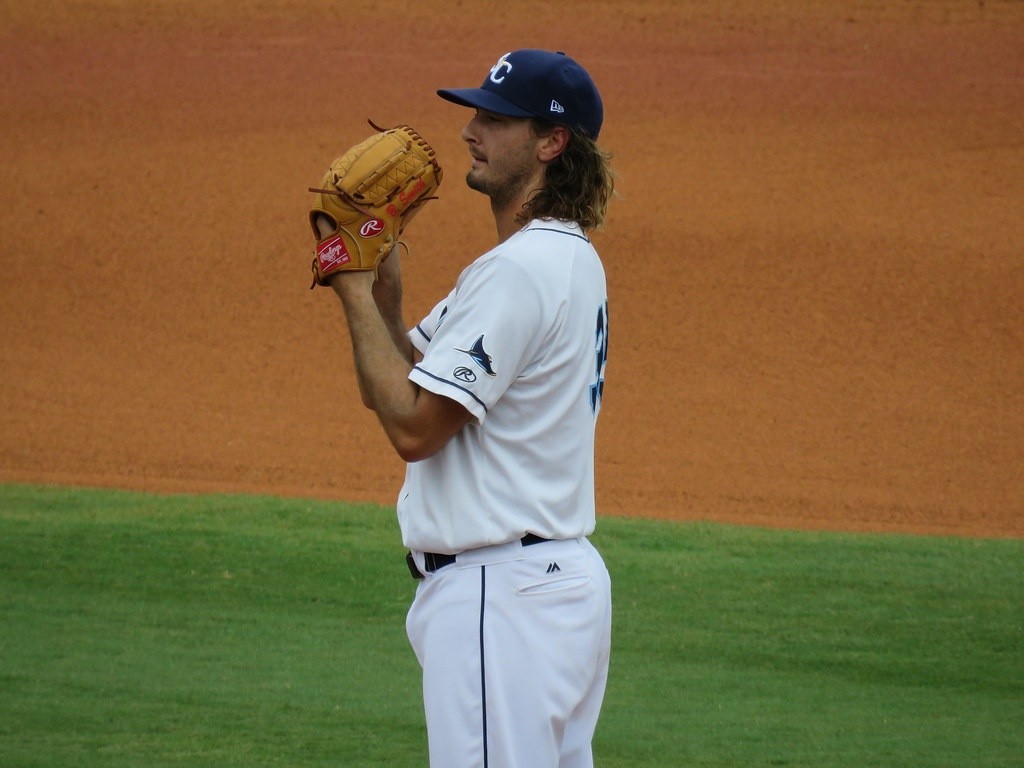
308 51 612 768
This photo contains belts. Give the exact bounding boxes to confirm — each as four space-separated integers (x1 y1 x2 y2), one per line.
407 531 548 582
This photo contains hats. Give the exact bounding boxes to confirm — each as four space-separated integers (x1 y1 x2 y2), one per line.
438 49 603 140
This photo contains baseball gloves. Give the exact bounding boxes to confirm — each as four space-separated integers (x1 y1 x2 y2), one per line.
306 124 450 282
